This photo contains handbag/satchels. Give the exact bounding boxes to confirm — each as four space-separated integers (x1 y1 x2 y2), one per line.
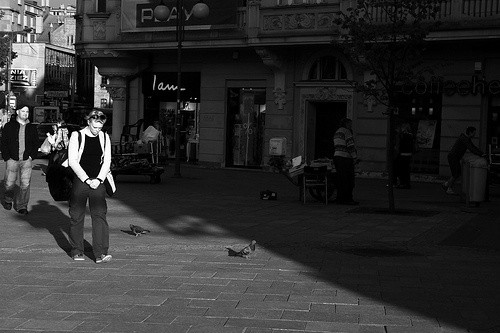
48 131 82 202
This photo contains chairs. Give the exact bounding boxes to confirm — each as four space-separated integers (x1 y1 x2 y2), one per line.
303 165 328 207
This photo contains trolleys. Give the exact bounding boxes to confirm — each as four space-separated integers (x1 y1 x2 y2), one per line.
271 154 338 202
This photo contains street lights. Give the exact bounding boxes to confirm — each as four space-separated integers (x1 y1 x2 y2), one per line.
4 34 12 93
154 0 209 178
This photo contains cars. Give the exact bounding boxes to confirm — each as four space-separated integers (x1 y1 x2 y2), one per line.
32 122 55 159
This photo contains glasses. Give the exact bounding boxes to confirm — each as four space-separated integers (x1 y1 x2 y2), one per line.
89 115 106 120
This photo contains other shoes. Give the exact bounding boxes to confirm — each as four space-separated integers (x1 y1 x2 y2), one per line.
3 202 12 210
447 189 459 195
19 209 28 214
345 201 360 205
73 254 85 260
95 254 112 263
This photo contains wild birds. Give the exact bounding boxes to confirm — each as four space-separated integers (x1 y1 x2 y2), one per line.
130 223 150 236
227 241 257 259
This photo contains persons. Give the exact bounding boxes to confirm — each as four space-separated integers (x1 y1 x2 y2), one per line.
333 118 360 205
0 105 38 214
441 127 489 195
62 111 116 262
42 120 69 176
386 149 413 189
